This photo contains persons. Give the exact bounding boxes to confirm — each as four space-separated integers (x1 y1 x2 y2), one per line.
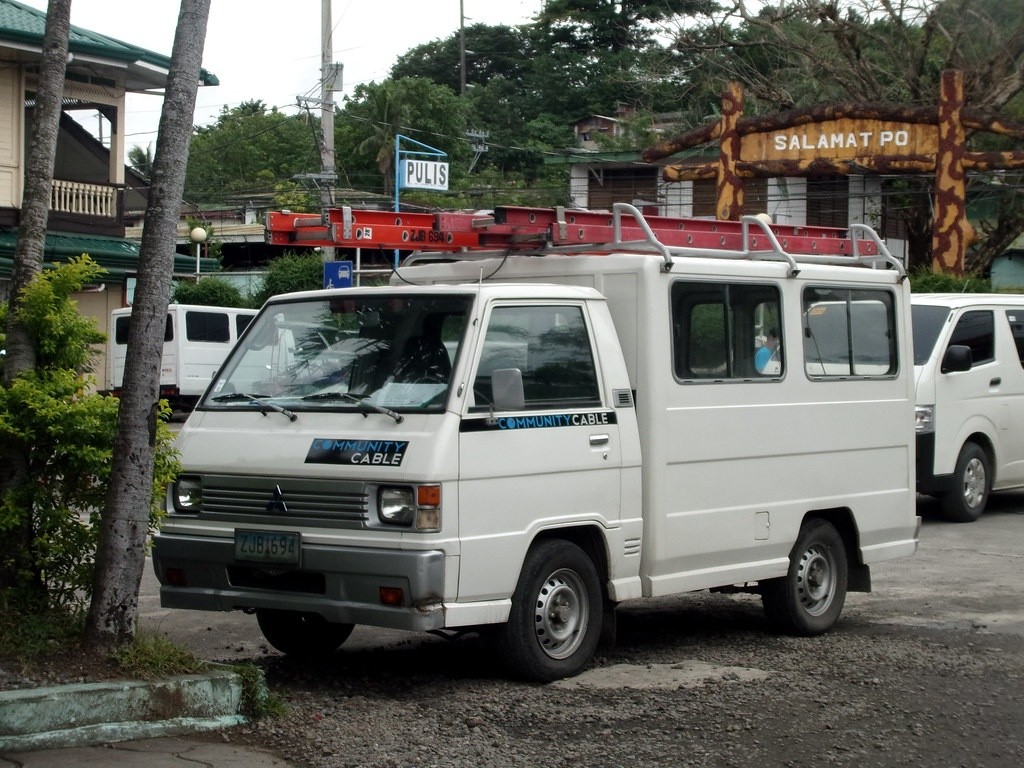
755 327 781 375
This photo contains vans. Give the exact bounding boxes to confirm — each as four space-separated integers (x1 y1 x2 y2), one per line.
109 302 303 418
756 292 1024 525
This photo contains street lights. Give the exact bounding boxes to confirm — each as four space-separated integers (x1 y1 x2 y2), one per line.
189 225 206 289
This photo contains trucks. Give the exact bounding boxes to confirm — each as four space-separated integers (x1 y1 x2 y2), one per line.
147 208 928 679
